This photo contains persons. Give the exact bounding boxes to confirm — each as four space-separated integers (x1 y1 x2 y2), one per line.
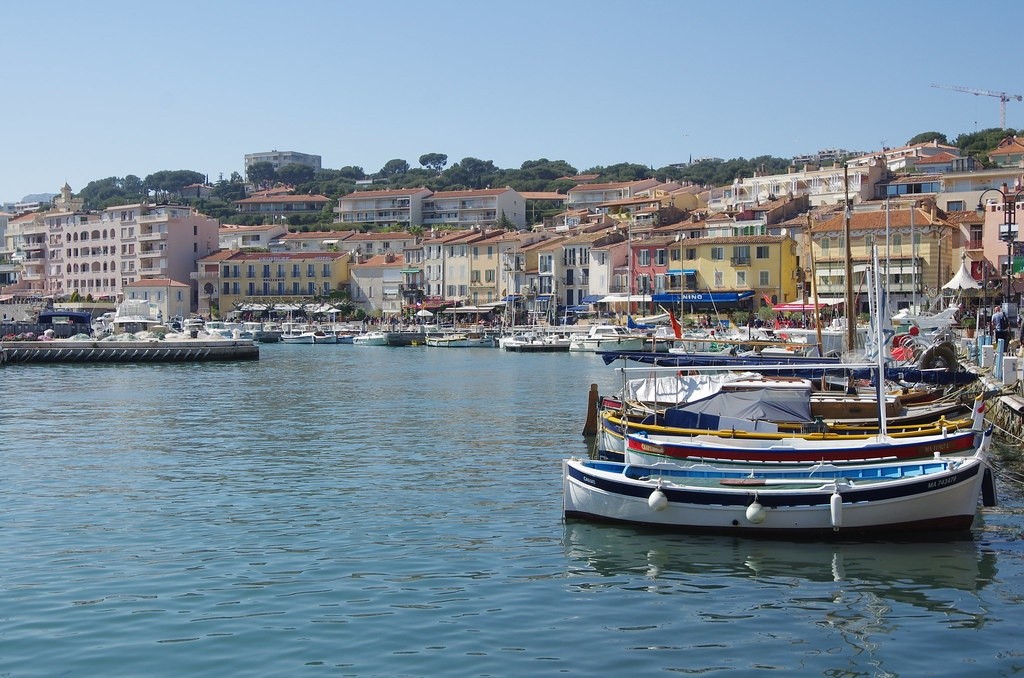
696 313 712 330
364 309 528 331
991 305 1009 354
771 311 831 331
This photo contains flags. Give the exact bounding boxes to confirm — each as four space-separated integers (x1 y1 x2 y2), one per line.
668 310 682 339
863 265 895 381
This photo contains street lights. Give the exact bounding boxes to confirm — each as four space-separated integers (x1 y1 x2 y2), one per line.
974 187 1024 350
976 256 996 330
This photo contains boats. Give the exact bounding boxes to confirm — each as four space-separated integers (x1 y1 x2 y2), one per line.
334 332 355 345
423 154 1001 540
351 329 390 347
279 301 339 344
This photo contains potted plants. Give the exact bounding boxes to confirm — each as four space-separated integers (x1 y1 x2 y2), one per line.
964 318 976 338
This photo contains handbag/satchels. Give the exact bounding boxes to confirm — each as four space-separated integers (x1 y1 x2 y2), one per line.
999 311 1009 331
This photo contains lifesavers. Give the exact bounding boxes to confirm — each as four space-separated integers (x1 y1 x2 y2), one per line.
919 346 958 374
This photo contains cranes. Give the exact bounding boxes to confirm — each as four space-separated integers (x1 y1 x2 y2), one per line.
927 82 1024 129
